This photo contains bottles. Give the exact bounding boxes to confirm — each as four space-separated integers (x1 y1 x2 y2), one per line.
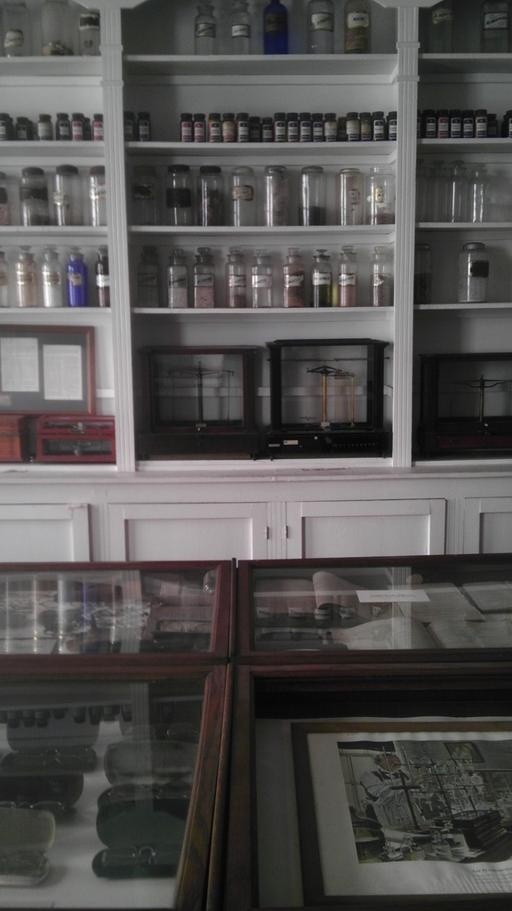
131 243 394 308
122 107 398 143
0 165 106 226
429 1 512 53
415 240 491 305
0 109 103 141
0 0 101 54
414 160 491 222
129 163 394 224
1 243 109 306
416 105 512 137
191 3 376 54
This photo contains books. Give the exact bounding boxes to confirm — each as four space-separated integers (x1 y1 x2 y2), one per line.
430 809 508 851
254 571 511 654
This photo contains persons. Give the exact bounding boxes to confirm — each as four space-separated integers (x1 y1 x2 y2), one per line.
361 753 421 848
